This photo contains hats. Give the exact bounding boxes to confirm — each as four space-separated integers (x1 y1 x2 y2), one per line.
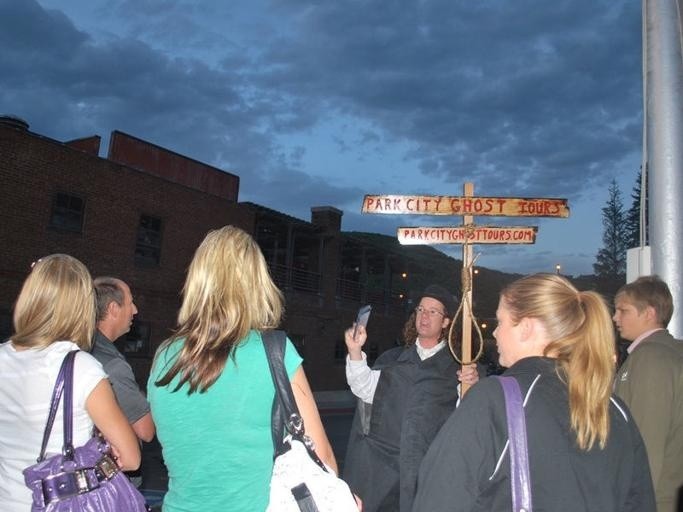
420 284 452 312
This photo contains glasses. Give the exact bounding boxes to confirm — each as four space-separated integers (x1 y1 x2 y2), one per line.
415 306 446 317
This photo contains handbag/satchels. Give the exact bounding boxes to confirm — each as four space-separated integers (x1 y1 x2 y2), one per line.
23 425 128 512
266 434 359 512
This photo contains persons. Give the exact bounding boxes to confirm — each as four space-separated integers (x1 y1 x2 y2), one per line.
147 224 364 512
344 284 486 512
412 273 658 511
87 276 156 489
610 274 682 511
1 253 141 511
